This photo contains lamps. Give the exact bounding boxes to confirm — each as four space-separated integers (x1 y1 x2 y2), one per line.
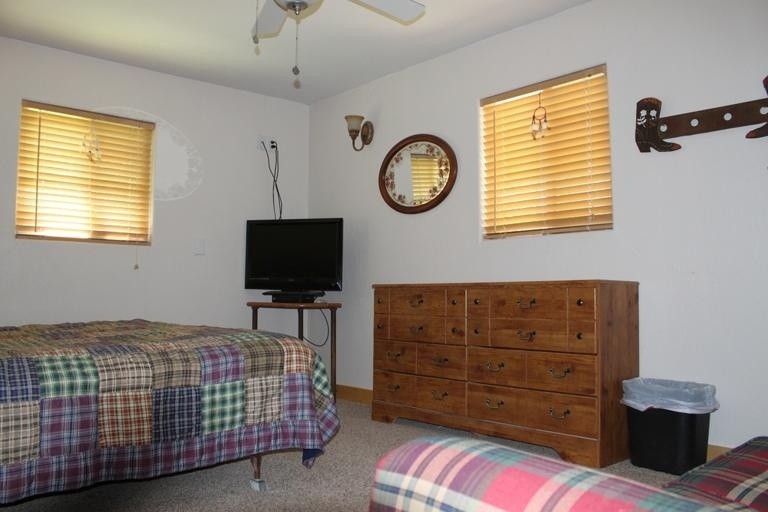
531 91 550 140
253 0 301 89
344 114 374 150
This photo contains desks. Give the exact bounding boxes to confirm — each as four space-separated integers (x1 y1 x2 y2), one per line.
246 301 341 409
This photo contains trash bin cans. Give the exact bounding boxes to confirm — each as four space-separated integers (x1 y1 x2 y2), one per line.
621 377 720 476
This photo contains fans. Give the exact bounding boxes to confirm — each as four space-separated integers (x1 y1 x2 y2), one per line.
251 0 425 36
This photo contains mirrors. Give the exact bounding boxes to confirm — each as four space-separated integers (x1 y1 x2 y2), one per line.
378 133 457 215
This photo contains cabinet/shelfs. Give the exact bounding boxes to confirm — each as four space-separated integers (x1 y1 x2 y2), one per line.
370 281 640 469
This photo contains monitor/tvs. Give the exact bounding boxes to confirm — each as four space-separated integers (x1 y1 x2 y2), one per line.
244 217 344 297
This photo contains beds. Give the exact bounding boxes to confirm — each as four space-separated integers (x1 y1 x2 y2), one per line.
0 318 339 505
367 433 768 512
